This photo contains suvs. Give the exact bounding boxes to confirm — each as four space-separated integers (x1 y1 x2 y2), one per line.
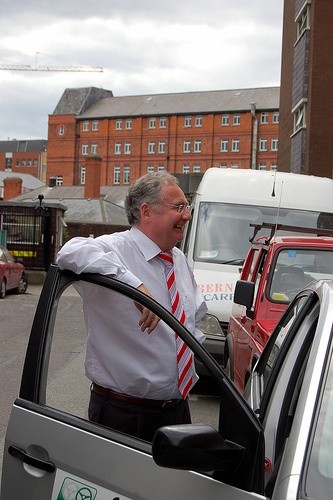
221 222 333 397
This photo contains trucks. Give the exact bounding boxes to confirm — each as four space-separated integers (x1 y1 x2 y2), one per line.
176 167 333 383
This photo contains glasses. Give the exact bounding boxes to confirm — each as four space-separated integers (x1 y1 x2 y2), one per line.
152 201 194 213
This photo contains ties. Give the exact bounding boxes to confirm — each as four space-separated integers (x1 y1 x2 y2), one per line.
156 253 193 400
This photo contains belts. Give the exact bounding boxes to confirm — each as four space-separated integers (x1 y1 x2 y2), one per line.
92 383 183 410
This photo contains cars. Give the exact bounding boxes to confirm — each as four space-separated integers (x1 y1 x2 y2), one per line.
0 262 333 499
0 246 29 298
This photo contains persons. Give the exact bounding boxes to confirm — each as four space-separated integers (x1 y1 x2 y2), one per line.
53 169 210 445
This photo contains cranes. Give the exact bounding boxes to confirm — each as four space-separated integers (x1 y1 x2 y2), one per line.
1 63 105 73
